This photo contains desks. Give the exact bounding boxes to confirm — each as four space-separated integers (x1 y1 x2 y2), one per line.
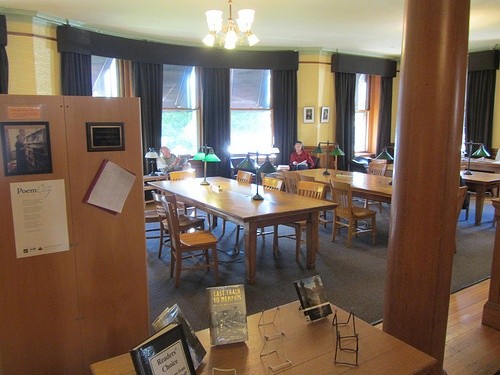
146 176 339 286
143 174 168 211
364 156 500 226
89 301 440 375
269 168 393 204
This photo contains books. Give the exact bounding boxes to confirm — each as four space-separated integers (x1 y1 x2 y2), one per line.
129 323 196 375
154 304 207 371
206 285 249 345
294 275 332 321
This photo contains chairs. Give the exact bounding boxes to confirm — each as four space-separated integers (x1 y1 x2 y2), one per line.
453 186 468 255
150 190 222 290
237 155 387 262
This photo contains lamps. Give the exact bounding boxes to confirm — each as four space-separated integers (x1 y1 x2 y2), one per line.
463 140 491 175
203 0 260 50
376 145 394 186
313 140 345 175
192 143 221 185
145 146 159 176
234 150 278 201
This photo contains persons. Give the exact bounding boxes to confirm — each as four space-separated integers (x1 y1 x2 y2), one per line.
156 147 186 174
289 140 314 171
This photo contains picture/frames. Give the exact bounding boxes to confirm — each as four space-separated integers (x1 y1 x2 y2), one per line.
304 106 314 124
320 106 330 124
0 121 53 176
86 122 125 152
